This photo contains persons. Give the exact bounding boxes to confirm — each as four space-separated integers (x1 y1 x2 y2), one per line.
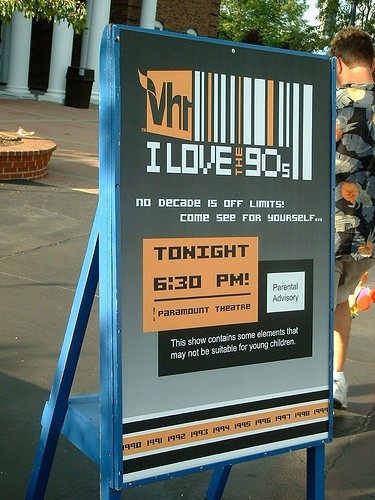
331 28 375 410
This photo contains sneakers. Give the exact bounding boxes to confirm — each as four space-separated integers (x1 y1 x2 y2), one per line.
333 379 349 409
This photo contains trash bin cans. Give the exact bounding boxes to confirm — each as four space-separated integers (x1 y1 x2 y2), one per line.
63 65 95 109
